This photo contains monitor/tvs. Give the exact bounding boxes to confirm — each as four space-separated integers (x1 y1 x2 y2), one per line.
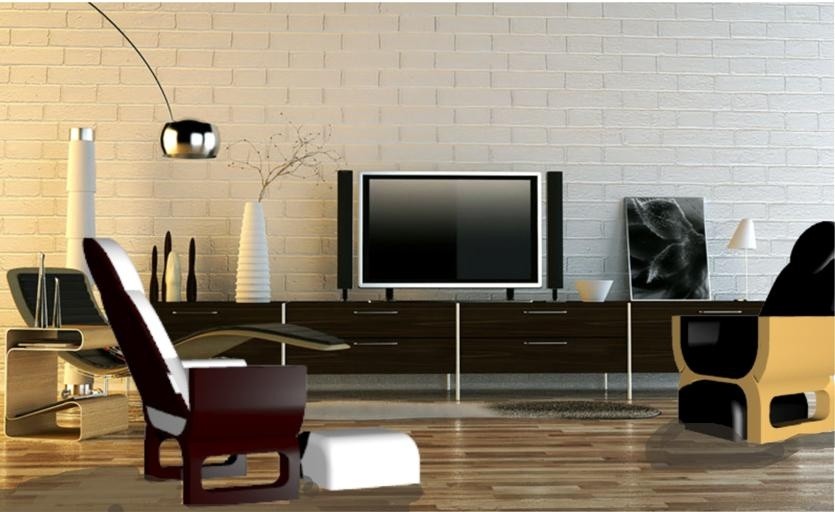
358 171 543 300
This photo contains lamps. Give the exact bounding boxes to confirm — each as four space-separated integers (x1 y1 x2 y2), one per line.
726 221 758 300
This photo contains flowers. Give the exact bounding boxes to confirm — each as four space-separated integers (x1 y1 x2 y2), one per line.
226 113 339 204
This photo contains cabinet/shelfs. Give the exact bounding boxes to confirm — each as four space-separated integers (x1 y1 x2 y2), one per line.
145 294 768 401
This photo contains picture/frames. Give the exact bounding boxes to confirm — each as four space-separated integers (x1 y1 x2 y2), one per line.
621 197 708 302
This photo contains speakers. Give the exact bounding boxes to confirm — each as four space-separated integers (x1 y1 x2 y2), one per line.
546 171 563 301
338 170 353 301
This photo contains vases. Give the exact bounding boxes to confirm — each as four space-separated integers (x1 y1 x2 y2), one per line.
234 202 273 306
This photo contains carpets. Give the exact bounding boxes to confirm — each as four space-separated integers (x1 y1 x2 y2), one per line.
304 396 663 419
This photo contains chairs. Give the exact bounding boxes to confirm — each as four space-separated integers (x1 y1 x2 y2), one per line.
670 220 833 446
